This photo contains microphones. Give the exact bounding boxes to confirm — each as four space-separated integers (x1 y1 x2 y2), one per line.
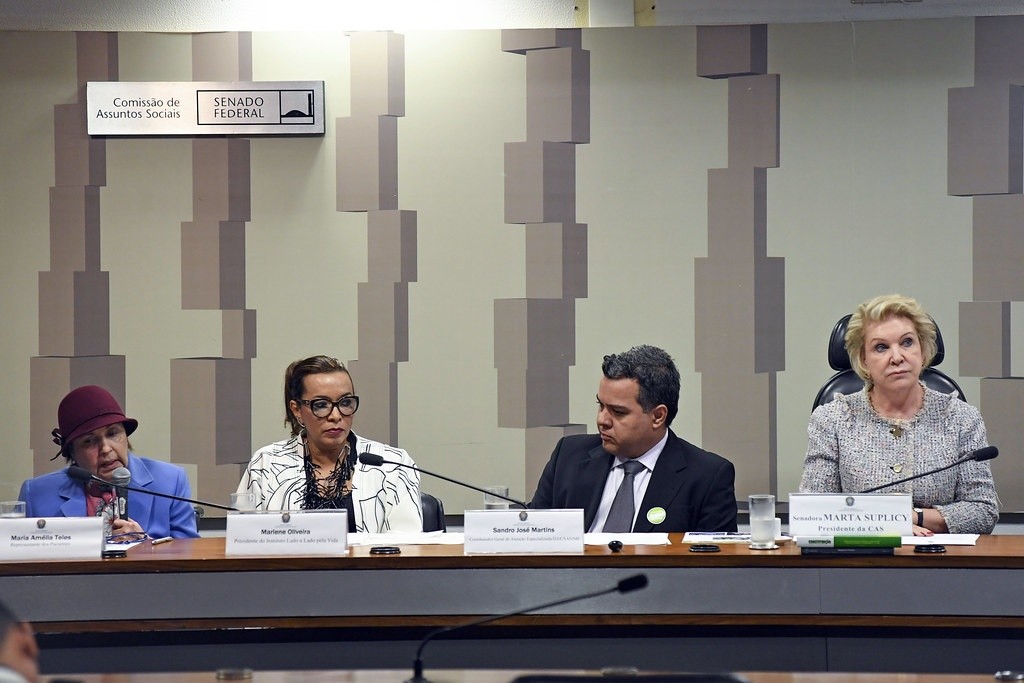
859 445 998 492
408 574 648 683
359 453 527 508
111 466 130 520
67 465 239 512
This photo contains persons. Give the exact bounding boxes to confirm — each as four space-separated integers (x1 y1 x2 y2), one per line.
236 356 423 533
799 294 1000 537
15 385 201 541
530 344 738 533
0 601 39 683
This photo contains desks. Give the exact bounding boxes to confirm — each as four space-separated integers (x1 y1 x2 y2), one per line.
0 535 1024 676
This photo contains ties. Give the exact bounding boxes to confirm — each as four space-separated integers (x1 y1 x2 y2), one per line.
602 461 645 533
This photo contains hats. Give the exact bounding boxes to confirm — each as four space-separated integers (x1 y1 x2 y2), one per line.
50 385 138 461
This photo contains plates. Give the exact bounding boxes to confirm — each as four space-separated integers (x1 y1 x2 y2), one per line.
775 536 792 543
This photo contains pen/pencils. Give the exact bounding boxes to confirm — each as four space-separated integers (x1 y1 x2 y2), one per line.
151 537 173 545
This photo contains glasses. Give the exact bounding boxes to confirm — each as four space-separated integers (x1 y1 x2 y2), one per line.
295 396 359 418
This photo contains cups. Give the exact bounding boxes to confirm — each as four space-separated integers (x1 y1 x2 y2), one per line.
0 501 26 518
230 492 256 512
748 495 775 549
775 517 781 540
483 485 510 510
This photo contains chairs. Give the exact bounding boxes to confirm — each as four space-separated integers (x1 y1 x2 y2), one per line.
810 312 968 413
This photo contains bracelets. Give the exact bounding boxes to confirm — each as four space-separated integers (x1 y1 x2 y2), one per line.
914 508 923 526
